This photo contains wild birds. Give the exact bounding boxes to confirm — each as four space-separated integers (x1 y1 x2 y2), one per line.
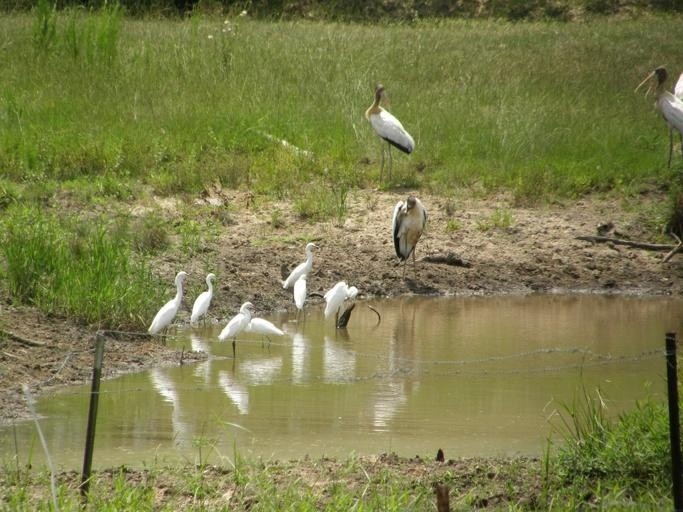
189 271 218 330
278 241 320 327
631 65 683 169
322 279 363 331
218 300 254 363
364 83 415 186
144 269 189 346
390 194 429 275
242 316 285 356
